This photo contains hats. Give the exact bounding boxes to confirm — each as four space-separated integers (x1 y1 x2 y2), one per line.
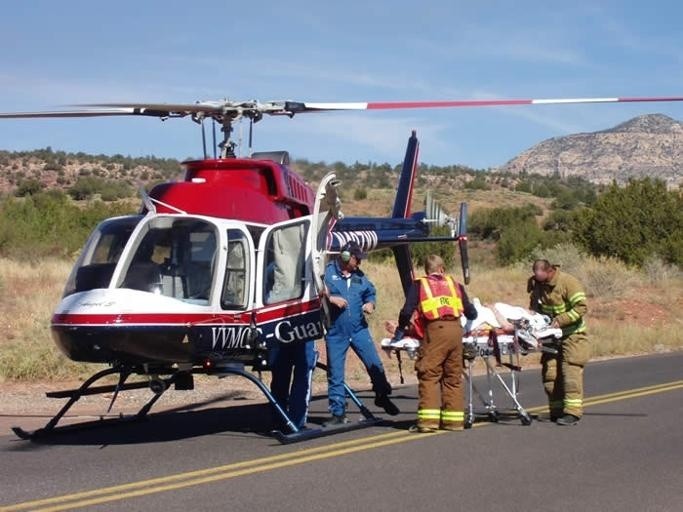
341 241 367 260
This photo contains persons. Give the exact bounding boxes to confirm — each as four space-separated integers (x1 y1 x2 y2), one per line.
393 252 479 433
264 254 329 434
384 302 537 334
526 258 590 425
322 241 402 427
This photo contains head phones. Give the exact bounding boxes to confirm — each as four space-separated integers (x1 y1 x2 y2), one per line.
341 241 353 262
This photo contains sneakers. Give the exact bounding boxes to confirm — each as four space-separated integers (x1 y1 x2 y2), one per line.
409 424 437 432
555 414 581 427
373 394 400 416
321 414 350 428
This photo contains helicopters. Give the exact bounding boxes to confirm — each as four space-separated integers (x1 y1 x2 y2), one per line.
0 96 683 445
